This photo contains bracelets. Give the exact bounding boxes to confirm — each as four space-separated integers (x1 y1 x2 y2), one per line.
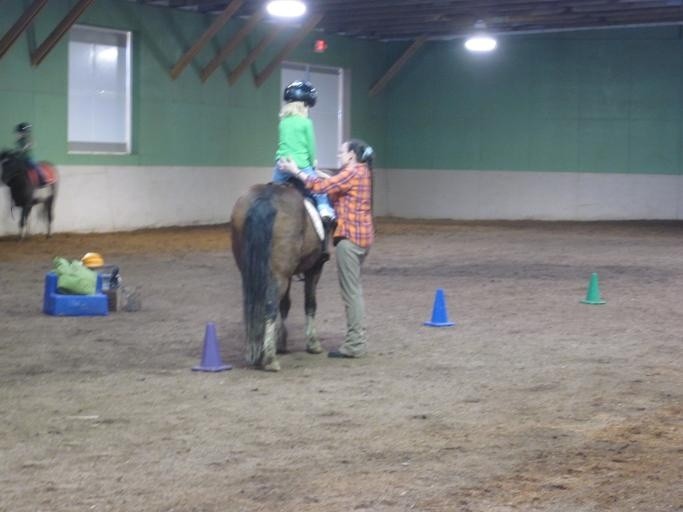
272 80 336 224
293 169 302 181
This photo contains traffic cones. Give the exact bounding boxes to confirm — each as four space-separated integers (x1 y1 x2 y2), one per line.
580 272 607 304
191 322 232 373
425 289 455 327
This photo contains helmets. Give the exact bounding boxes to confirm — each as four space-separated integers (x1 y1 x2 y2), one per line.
16 123 31 132
284 80 316 106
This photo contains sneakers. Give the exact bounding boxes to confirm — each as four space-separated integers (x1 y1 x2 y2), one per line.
323 217 338 229
329 351 356 358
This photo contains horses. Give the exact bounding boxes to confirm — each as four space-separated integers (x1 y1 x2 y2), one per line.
230 168 341 371
0 150 58 243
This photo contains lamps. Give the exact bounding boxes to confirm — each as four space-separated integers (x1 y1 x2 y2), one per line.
460 18 499 55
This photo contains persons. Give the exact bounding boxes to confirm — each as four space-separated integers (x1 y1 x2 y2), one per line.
277 138 375 358
14 122 50 187
